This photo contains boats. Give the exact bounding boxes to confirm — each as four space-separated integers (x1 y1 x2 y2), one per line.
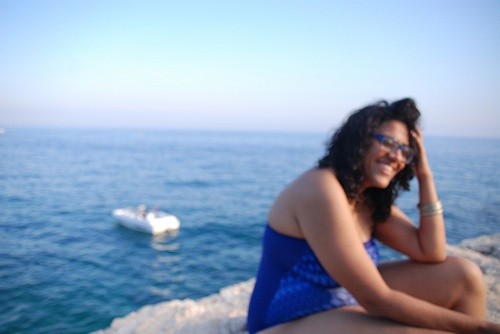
112 205 181 235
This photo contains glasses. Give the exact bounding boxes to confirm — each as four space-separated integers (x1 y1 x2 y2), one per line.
371 132 414 159
